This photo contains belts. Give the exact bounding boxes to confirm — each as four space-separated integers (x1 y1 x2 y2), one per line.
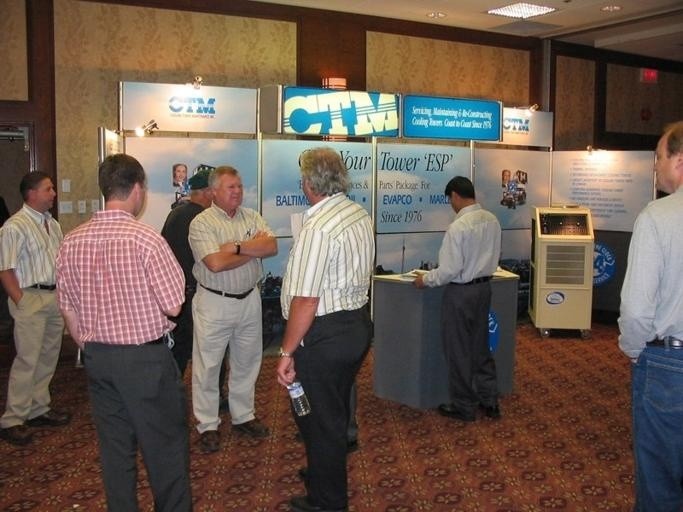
448 275 490 286
200 283 256 299
646 336 683 349
145 335 168 346
29 284 58 291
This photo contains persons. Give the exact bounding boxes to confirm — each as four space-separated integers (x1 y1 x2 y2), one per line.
616 117 683 512
501 170 511 188
188 165 279 453
1 170 70 445
54 153 193 512
412 175 502 422
158 170 230 417
276 146 375 512
172 163 188 187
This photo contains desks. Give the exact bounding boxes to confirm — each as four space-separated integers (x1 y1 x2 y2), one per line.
372 266 518 417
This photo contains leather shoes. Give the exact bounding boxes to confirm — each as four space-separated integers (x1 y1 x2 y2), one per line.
25 409 72 427
231 417 270 436
0 423 34 445
287 495 349 512
437 402 476 422
475 395 501 418
200 428 221 452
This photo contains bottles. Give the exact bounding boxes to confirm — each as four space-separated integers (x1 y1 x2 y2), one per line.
285 372 312 418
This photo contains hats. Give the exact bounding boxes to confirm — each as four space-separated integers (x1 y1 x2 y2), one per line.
187 170 212 190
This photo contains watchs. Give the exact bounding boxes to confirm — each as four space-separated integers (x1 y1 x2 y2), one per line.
234 239 242 255
